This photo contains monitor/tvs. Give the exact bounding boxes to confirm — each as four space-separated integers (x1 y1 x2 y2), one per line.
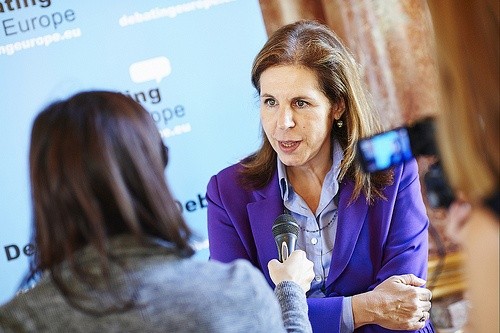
360 127 412 173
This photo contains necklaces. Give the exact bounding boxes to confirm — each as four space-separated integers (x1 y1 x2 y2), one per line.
297 211 337 232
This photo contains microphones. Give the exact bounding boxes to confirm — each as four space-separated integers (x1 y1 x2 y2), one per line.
271 214 300 263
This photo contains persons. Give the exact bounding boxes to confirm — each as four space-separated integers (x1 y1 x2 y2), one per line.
205 21 434 333
423 0 500 332
0 90 315 333
